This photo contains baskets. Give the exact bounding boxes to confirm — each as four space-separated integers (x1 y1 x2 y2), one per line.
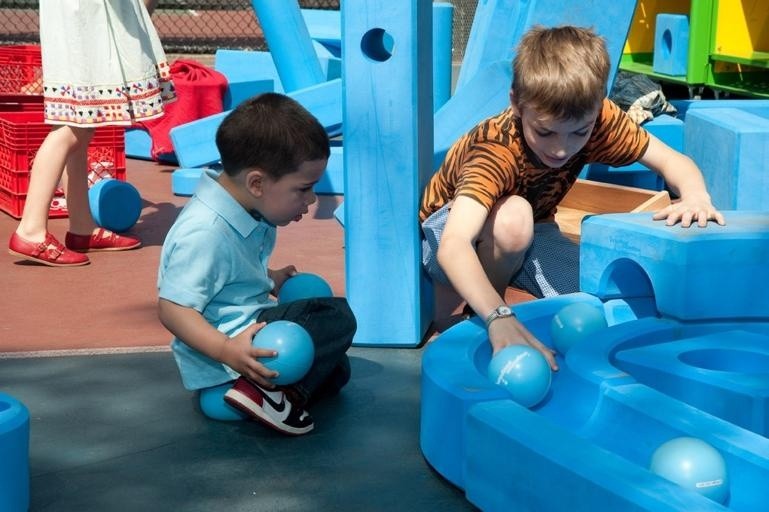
0 44 126 219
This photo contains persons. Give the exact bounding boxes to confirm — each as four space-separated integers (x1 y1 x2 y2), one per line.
7 0 179 268
154 93 360 437
415 22 729 372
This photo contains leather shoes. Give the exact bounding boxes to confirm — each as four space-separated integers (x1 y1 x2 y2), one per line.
7 230 91 268
66 228 143 253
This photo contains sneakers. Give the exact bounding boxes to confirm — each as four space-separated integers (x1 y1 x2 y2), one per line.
223 375 315 435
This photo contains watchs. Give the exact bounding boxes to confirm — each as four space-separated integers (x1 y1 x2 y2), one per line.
486 304 516 330
